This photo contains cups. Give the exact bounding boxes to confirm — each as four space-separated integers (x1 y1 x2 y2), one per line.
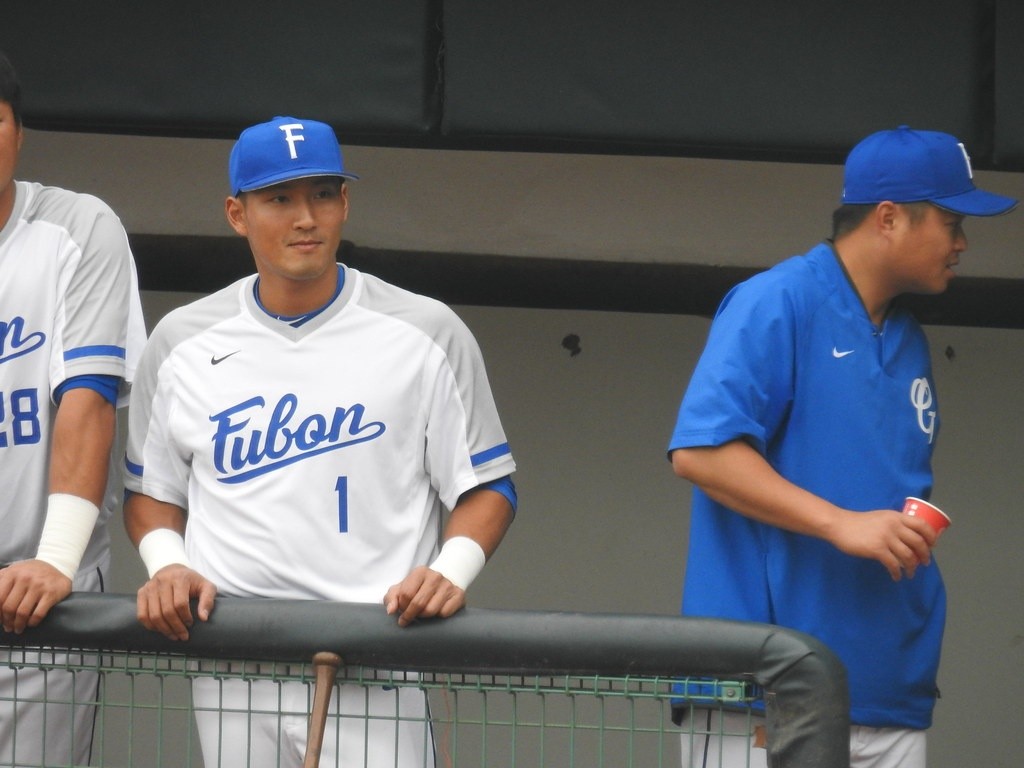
879 497 952 568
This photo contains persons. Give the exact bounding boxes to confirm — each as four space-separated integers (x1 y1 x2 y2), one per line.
118 115 519 768
0 63 144 768
667 124 1023 768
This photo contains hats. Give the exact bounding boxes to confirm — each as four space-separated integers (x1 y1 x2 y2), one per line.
841 125 1020 218
229 116 359 199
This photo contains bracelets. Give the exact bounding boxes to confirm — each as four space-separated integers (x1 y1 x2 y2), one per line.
35 494 101 581
139 529 189 579
430 536 485 593
